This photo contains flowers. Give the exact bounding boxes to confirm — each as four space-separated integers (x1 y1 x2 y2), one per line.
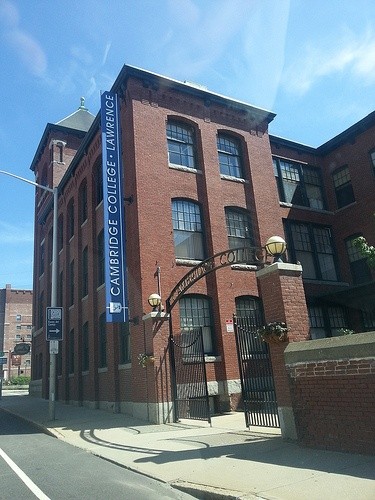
137 352 147 368
254 321 291 339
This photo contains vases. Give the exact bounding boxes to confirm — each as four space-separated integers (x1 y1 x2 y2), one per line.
262 332 287 345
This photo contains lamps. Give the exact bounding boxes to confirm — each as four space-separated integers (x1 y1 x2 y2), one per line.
265 235 287 263
148 294 162 312
